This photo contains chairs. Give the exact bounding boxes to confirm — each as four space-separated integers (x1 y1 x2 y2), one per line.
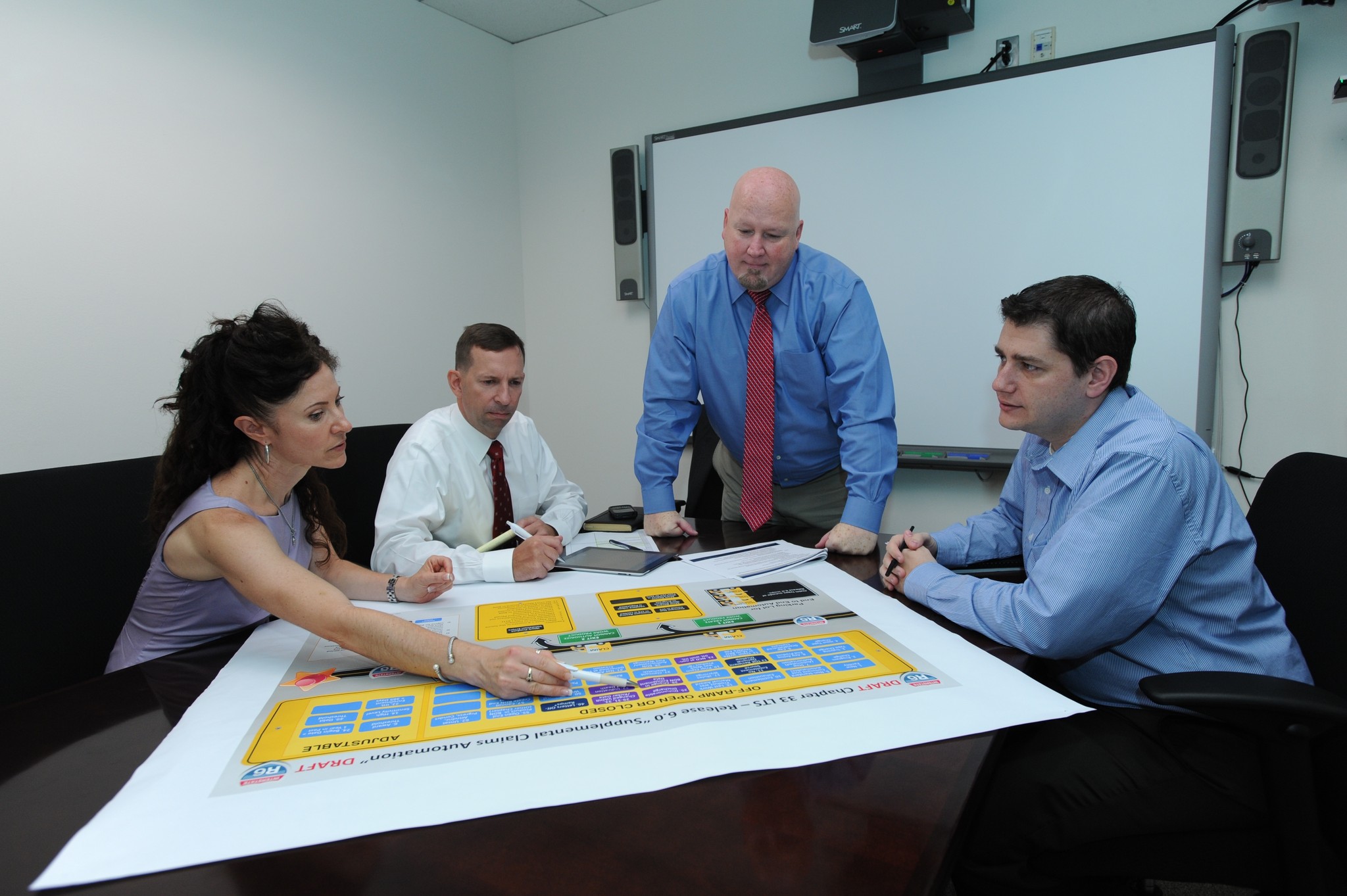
316 422 414 574
1069 448 1346 896
1 453 161 704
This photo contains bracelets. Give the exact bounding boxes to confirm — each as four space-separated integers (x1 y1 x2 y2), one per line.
433 636 457 684
385 574 402 603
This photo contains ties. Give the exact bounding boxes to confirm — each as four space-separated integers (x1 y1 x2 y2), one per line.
742 286 779 533
487 443 515 557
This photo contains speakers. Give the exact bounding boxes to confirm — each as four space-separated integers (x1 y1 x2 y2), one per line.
1220 20 1301 265
609 144 646 302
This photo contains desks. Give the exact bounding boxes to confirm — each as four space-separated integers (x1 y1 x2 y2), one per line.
0 516 1041 895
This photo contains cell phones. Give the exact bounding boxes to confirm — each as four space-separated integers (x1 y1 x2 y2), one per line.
608 505 639 520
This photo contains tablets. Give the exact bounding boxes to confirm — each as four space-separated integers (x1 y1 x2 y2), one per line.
555 546 680 577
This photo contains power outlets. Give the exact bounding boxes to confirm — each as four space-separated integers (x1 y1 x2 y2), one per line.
996 34 1019 70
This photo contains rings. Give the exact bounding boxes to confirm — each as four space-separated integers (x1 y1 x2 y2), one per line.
535 649 542 655
526 666 533 682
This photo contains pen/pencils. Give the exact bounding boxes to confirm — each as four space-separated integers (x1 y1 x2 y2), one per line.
884 525 915 577
506 521 566 563
557 662 640 687
608 540 644 551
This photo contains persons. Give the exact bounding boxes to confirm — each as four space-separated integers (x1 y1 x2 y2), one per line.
877 276 1329 896
370 322 590 586
104 302 572 702
633 167 898 556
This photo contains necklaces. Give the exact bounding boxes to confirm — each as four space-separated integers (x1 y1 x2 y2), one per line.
243 455 298 547
